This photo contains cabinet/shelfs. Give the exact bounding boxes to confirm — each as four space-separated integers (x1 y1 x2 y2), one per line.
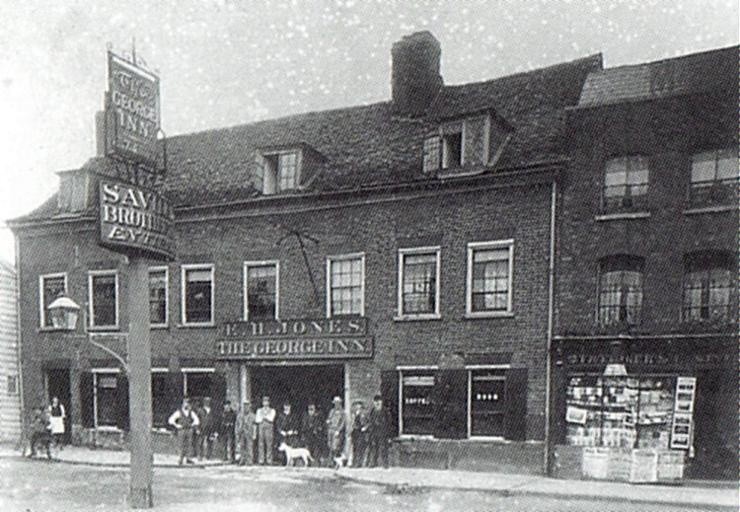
562 373 680 452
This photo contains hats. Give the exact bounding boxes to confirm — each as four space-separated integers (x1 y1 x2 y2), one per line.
332 395 343 403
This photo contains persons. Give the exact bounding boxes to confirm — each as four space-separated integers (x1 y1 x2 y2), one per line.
27 403 53 460
166 394 395 470
45 394 67 450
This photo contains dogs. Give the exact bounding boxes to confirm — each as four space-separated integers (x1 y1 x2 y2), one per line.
277 442 314 467
